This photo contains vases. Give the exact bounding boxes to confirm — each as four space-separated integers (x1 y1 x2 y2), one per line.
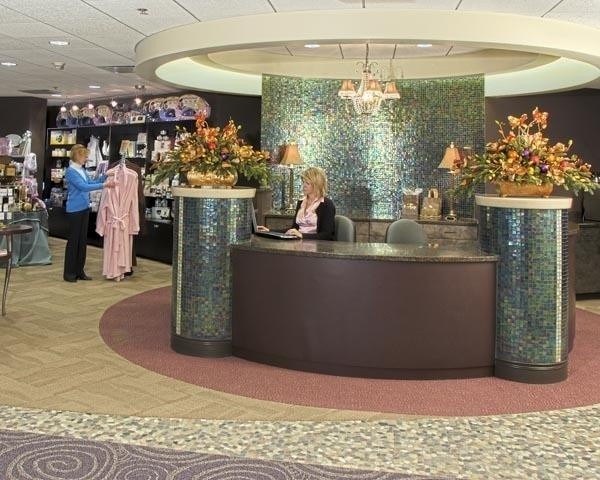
494 178 554 199
187 162 238 189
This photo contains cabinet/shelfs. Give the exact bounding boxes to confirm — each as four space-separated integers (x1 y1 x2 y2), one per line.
41 119 213 266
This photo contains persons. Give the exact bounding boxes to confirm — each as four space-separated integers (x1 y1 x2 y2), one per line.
63 143 117 283
257 166 337 240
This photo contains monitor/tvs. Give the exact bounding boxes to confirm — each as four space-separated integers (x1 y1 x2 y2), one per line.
251 203 257 233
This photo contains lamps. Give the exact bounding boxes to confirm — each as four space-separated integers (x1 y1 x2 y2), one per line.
280 142 306 214
337 44 401 116
437 142 463 221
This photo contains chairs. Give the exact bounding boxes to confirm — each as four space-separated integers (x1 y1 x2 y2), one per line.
385 219 429 243
334 214 357 243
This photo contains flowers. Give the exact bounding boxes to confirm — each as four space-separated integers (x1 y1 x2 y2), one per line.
146 111 283 191
444 106 600 205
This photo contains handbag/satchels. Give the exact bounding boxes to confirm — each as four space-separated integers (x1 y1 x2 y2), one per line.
422 188 442 215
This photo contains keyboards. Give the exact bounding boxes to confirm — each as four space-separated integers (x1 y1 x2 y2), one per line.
257 232 299 240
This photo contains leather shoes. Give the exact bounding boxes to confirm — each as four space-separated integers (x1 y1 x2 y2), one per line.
83 274 92 280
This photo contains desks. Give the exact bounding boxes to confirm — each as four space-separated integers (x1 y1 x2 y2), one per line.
0 224 34 316
